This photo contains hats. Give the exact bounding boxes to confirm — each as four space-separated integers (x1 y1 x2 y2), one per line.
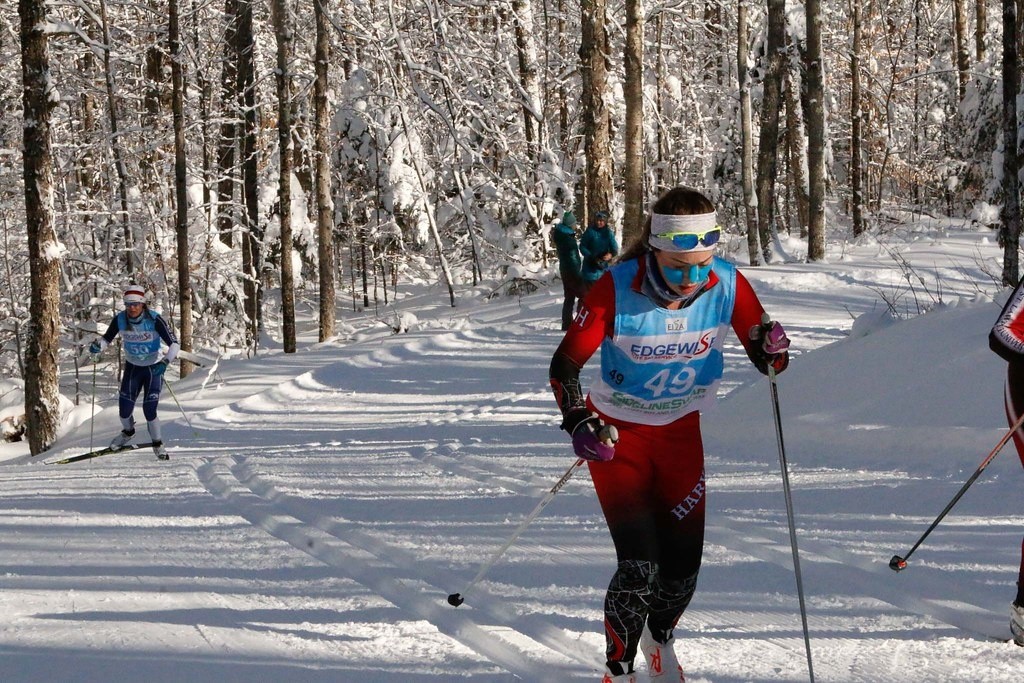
123 285 147 303
561 209 577 227
594 210 610 218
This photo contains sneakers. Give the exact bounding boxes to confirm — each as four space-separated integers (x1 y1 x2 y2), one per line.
600 671 638 683
641 622 685 683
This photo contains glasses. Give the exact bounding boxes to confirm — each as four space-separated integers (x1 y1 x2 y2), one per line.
649 225 723 250
124 301 141 307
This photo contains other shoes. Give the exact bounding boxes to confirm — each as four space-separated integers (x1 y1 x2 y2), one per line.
151 443 169 460
1009 600 1024 647
562 326 568 331
110 429 136 451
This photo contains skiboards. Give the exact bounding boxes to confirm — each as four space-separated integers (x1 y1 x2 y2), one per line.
43 442 170 466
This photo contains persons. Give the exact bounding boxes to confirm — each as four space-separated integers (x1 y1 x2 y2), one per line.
988 271 1024 648
579 211 618 289
90 284 179 461
554 209 583 331
547 184 790 683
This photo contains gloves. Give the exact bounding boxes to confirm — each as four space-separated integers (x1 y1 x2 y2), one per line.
559 406 615 461
749 321 791 362
588 255 600 265
89 341 101 353
149 357 170 376
